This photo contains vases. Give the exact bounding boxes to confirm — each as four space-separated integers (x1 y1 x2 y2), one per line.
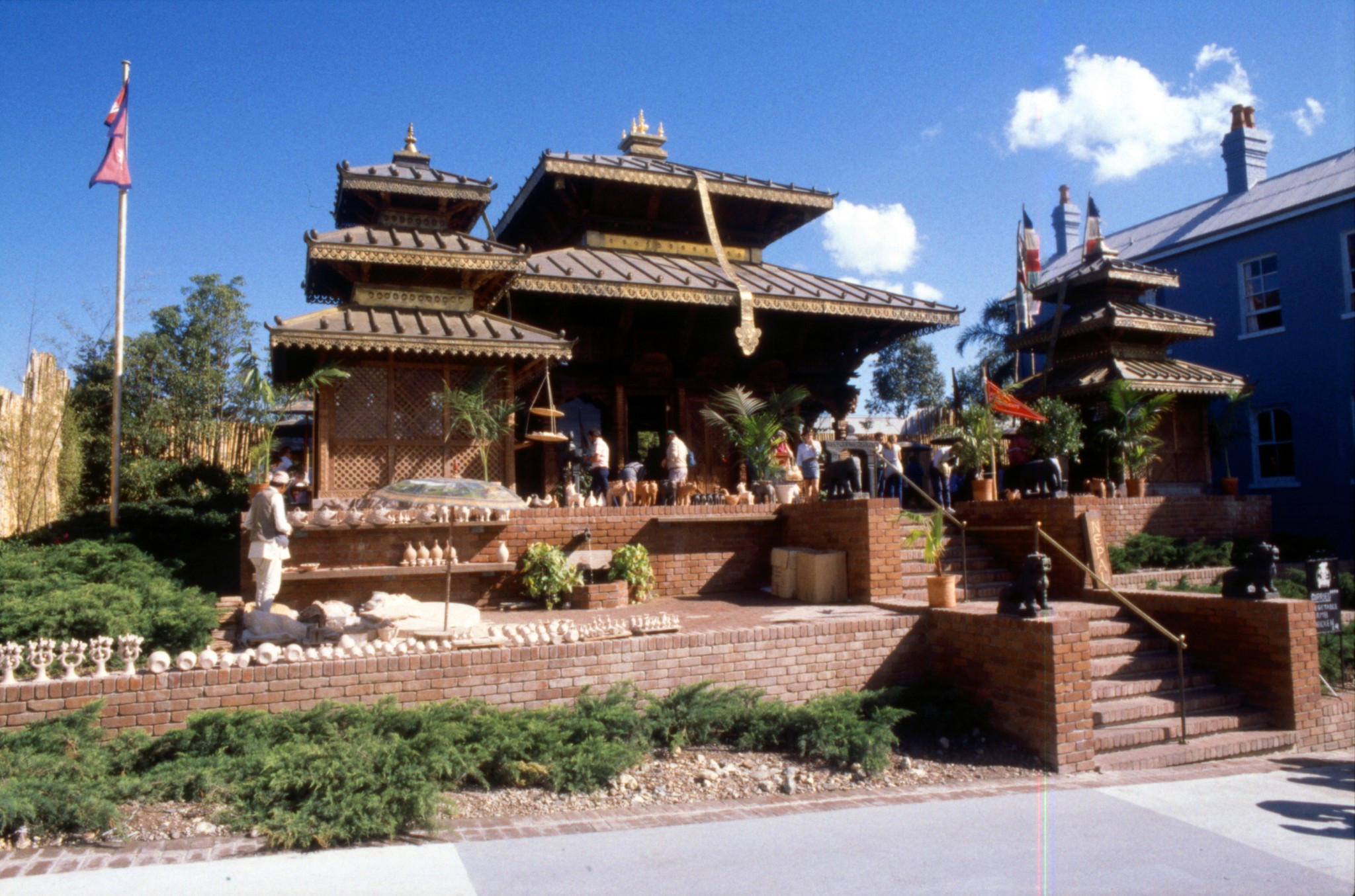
772 478 800 506
1090 477 1106 498
1104 479 1117 499
1083 477 1090 495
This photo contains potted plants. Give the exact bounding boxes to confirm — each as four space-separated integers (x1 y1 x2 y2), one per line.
1203 385 1254 494
896 503 957 607
700 379 811 505
1016 396 1087 492
227 340 350 507
934 395 1009 500
1096 377 1176 497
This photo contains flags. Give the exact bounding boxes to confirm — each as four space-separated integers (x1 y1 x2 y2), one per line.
988 380 1050 421
87 76 132 190
1016 208 1040 293
1086 197 1101 259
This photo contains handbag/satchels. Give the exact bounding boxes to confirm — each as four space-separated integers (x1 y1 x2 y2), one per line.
784 457 803 481
686 446 696 466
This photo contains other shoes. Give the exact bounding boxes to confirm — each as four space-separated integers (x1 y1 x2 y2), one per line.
947 507 956 514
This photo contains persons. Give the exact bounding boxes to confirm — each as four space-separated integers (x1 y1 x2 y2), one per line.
268 444 310 505
662 429 689 504
868 431 965 513
244 470 294 614
583 429 619 507
774 427 823 502
617 461 648 503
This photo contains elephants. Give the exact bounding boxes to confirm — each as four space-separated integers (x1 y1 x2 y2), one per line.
824 456 862 499
1003 457 1063 499
606 478 698 508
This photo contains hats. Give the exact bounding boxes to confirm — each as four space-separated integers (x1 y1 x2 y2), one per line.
776 429 786 438
664 429 675 436
270 470 290 484
